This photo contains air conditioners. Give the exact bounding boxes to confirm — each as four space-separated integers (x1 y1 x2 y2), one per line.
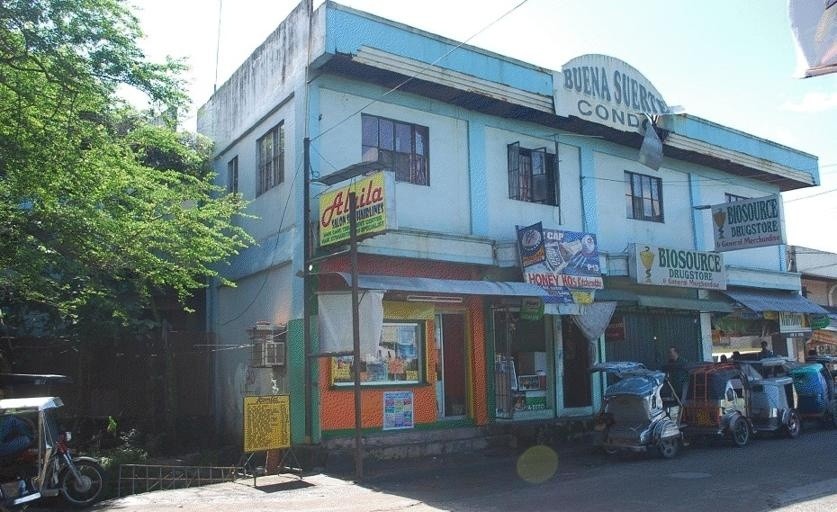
250 324 287 369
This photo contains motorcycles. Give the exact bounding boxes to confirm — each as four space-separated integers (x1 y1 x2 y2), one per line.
0 396 110 512
590 356 837 459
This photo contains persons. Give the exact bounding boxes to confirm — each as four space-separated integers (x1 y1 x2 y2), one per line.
759 341 772 361
659 345 695 406
